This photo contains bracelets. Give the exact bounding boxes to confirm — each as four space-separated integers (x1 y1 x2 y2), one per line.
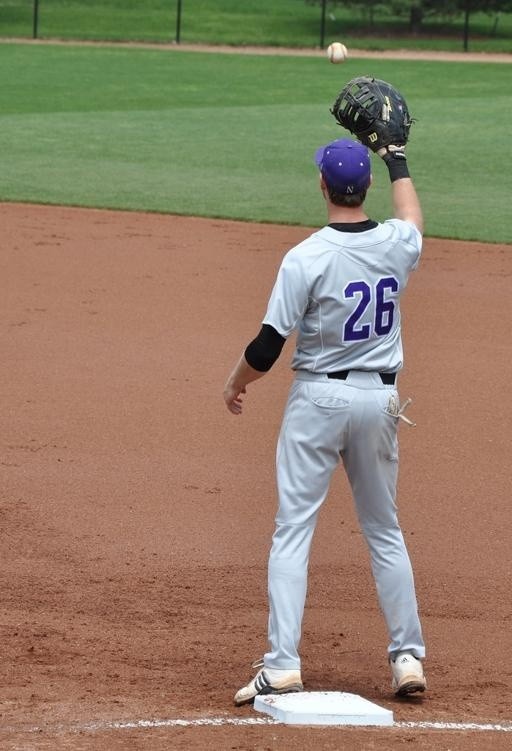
382 151 411 183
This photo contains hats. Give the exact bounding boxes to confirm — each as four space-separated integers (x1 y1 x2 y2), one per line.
316 138 371 194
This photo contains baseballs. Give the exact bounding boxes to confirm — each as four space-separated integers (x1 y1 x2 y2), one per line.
327 40 349 64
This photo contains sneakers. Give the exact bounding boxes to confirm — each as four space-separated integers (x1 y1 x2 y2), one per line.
233 665 304 706
389 653 426 696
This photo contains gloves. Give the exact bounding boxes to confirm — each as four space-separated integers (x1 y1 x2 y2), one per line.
384 396 417 429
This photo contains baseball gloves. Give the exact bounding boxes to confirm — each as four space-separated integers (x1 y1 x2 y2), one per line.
330 76 414 159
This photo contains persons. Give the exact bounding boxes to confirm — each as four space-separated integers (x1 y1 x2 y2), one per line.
222 74 429 706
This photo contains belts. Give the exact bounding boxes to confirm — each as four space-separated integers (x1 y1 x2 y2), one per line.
327 367 397 386
